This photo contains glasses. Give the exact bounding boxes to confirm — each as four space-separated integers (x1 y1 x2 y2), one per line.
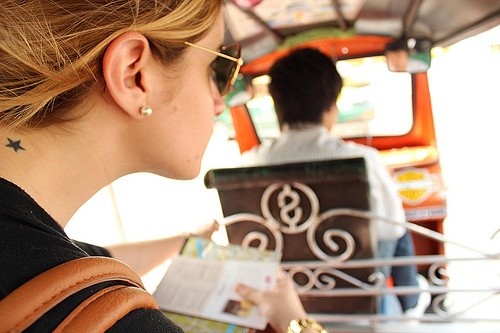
182 41 245 95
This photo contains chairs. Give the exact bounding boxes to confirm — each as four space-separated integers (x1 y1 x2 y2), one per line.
205 157 377 314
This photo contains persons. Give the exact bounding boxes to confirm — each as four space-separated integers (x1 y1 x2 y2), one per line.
236 45 433 321
385 41 430 75
0 1 331 333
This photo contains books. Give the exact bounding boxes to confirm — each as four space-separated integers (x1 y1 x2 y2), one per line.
152 234 283 333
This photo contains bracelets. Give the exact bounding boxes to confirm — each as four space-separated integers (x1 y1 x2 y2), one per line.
287 319 328 333
